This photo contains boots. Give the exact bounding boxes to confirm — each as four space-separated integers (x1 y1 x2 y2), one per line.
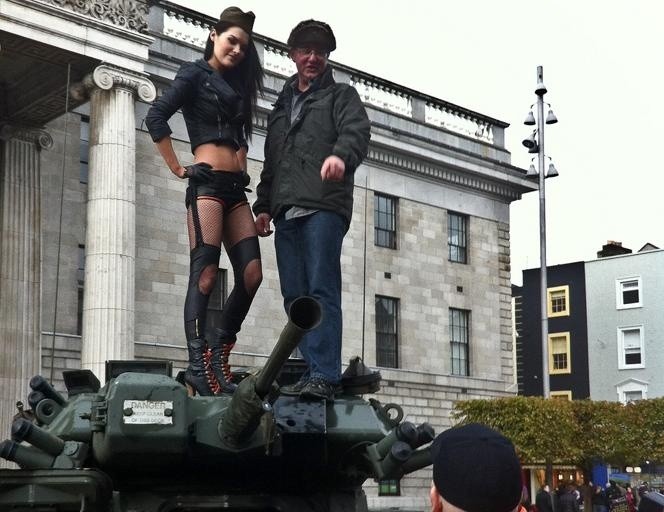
185 328 238 396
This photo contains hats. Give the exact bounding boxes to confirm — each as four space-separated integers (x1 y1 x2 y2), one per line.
288 20 336 51
220 8 255 34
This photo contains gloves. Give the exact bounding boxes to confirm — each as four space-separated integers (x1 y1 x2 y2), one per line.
183 163 216 183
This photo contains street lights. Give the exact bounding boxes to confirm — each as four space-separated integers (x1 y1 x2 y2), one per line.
521 64 562 403
625 463 641 487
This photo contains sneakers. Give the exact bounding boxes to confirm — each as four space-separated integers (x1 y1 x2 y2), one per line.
279 377 342 401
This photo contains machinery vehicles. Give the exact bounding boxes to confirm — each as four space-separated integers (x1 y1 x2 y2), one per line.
0 333 436 511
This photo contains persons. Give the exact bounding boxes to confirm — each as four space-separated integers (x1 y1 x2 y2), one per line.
144 6 268 396
535 478 664 512
251 19 372 400
